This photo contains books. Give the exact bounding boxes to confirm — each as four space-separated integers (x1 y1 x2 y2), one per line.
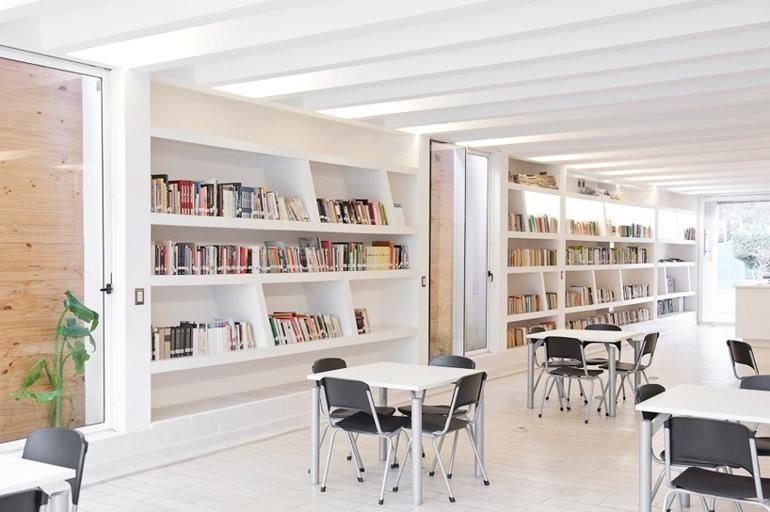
268 312 342 346
667 277 676 293
257 237 409 272
546 292 557 310
683 226 695 241
597 287 616 304
507 320 556 348
150 174 310 221
355 308 371 334
613 307 651 326
508 211 557 233
508 294 542 314
565 286 593 307
567 219 601 235
566 245 609 265
606 219 651 238
658 299 673 316
508 170 557 189
152 240 253 274
611 246 648 264
508 248 555 267
317 198 389 226
622 283 650 300
565 313 608 329
152 320 256 361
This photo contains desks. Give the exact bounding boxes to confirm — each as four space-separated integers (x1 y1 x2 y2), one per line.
0 453 76 512
526 330 643 416
636 384 770 511
305 359 488 503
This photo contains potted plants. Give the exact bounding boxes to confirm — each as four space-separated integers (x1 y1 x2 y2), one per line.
13 290 98 427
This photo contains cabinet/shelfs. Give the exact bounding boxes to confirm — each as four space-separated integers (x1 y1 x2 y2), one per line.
501 154 698 349
151 127 420 375
734 282 770 340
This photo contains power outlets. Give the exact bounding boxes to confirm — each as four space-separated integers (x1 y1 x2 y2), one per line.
135 288 143 305
423 276 426 286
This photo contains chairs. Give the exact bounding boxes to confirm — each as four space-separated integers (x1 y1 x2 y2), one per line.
0 429 87 512
727 341 770 391
637 385 744 510
319 377 412 504
580 324 626 402
393 371 489 501
307 359 395 472
392 356 477 479
598 333 659 412
664 418 769 512
539 337 609 423
527 327 588 410
755 438 770 456
